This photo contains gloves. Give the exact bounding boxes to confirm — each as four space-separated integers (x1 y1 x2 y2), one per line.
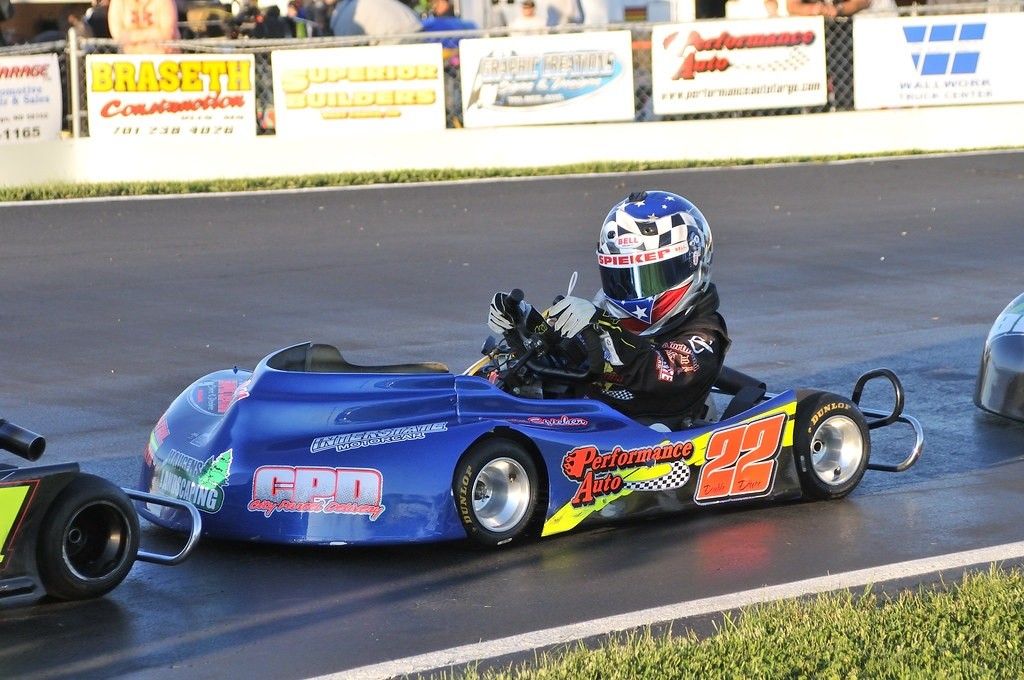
487 291 531 334
548 296 605 338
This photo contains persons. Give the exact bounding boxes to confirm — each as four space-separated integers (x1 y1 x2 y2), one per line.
68 0 112 38
787 0 872 113
764 0 784 19
488 190 733 432
225 0 547 40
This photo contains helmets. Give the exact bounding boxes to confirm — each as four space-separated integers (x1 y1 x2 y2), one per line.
596 190 713 338
